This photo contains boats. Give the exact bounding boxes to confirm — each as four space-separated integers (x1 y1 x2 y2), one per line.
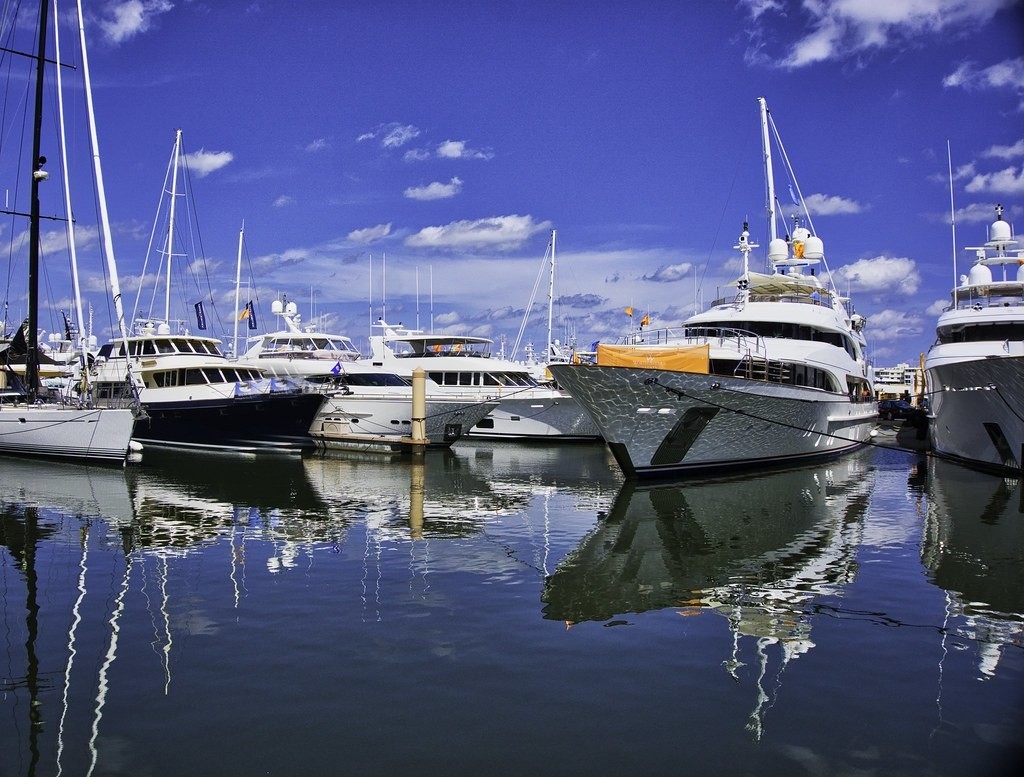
545 94 882 491
921 138 1024 480
0 0 605 466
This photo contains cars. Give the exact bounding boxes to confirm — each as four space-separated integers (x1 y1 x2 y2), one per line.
878 399 916 421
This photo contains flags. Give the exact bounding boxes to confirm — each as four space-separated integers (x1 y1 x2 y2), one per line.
11 323 30 356
237 309 249 321
332 363 342 375
245 300 258 329
641 316 648 325
624 305 634 317
432 344 443 352
454 344 463 350
195 301 207 330
